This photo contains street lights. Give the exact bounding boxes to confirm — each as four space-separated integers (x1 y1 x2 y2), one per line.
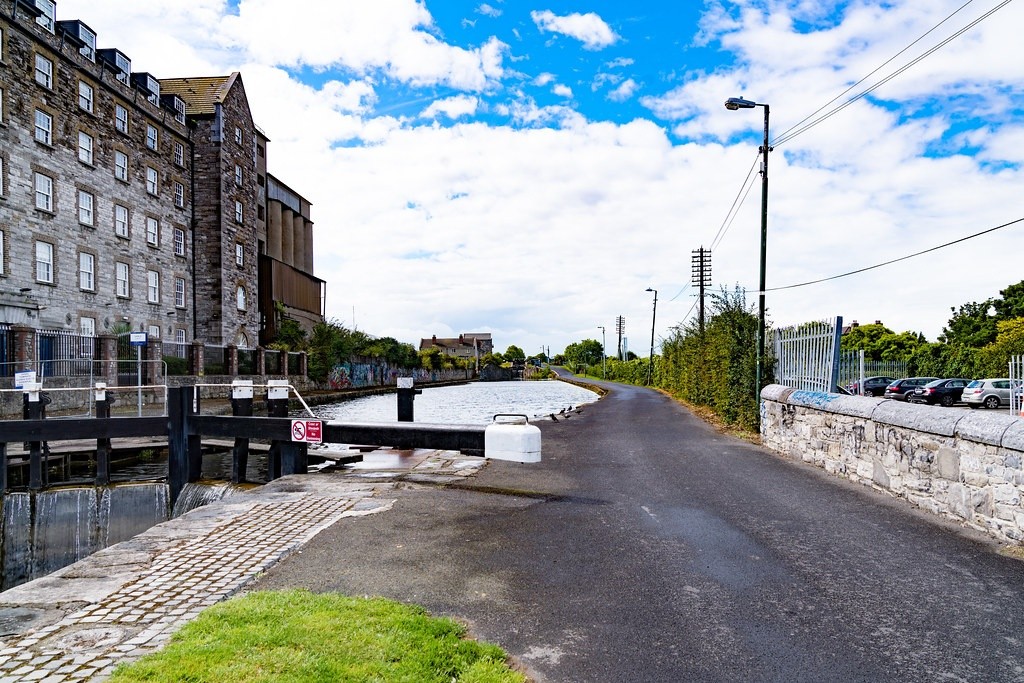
598 326 606 379
646 287 658 386
725 96 768 432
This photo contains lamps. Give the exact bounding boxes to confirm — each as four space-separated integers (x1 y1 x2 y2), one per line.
167 312 175 315
19 288 32 291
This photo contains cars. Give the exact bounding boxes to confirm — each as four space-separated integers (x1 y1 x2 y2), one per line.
914 379 973 407
961 379 1023 409
885 377 939 400
848 376 897 397
